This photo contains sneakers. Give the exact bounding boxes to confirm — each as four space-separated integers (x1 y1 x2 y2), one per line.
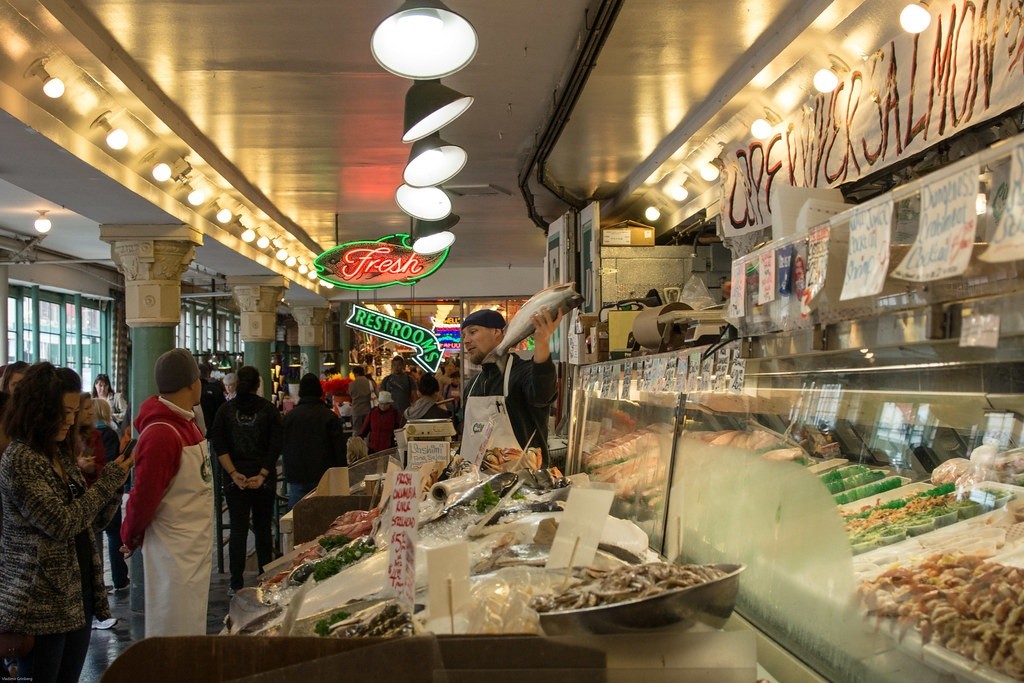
117 579 131 590
228 585 238 596
105 586 113 592
92 615 118 630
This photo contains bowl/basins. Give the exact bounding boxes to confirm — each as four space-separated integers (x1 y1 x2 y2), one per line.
851 488 1019 554
538 562 746 634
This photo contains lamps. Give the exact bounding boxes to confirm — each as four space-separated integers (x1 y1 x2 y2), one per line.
288 353 302 368
323 352 335 366
218 354 231 371
368 0 479 256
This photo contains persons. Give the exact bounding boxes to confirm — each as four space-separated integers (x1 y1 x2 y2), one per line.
75 392 118 630
91 374 127 442
213 366 283 597
191 354 243 507
282 373 344 512
320 348 461 475
0 361 30 683
120 348 214 639
0 361 135 683
794 256 806 301
451 308 562 469
92 399 130 594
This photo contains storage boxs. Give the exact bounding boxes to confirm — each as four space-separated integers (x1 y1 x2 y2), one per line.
601 220 655 246
568 308 608 365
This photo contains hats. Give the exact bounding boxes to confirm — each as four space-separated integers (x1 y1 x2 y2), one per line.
298 373 323 399
155 348 199 394
377 391 394 403
461 309 506 329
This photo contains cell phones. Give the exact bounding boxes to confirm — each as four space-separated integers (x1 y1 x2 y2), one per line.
122 439 137 462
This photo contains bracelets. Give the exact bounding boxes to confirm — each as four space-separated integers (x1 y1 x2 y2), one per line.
260 472 267 478
229 470 236 474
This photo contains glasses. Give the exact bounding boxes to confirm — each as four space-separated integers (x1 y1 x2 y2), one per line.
97 374 109 380
